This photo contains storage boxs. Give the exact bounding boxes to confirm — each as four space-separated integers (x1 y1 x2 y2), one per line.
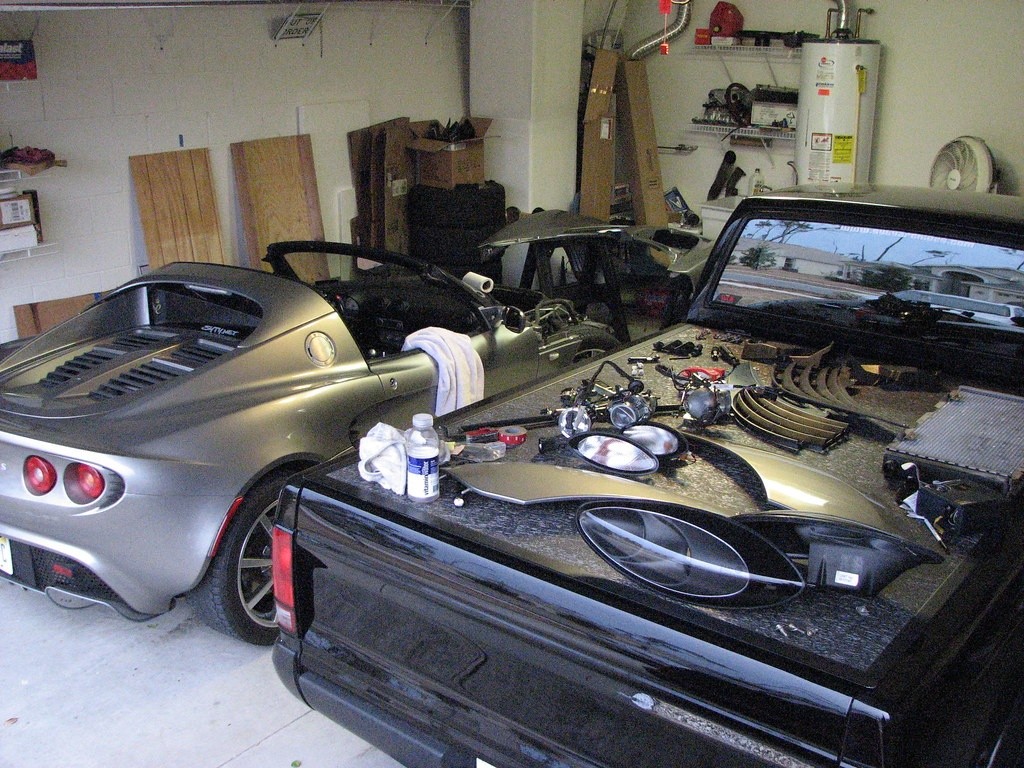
574 51 617 224
618 60 669 227
348 118 412 267
408 116 493 189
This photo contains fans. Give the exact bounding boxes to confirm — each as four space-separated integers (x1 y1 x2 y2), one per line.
931 136 997 193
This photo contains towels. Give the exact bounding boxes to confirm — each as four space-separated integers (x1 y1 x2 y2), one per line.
357 422 451 496
401 326 486 419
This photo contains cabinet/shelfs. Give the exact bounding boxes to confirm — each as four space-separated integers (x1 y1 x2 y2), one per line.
701 194 744 239
684 46 801 140
0 172 56 261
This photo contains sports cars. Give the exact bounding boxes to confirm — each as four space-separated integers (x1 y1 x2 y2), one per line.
0 235 641 649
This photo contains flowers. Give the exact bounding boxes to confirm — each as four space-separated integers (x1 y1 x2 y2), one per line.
5 147 54 165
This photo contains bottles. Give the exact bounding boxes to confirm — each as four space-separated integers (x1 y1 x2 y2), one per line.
405 412 441 504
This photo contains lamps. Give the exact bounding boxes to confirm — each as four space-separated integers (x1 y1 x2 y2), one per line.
272 1 304 47
427 1 456 43
304 3 335 45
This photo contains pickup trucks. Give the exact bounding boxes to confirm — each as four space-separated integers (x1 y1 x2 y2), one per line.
276 182 1022 768
